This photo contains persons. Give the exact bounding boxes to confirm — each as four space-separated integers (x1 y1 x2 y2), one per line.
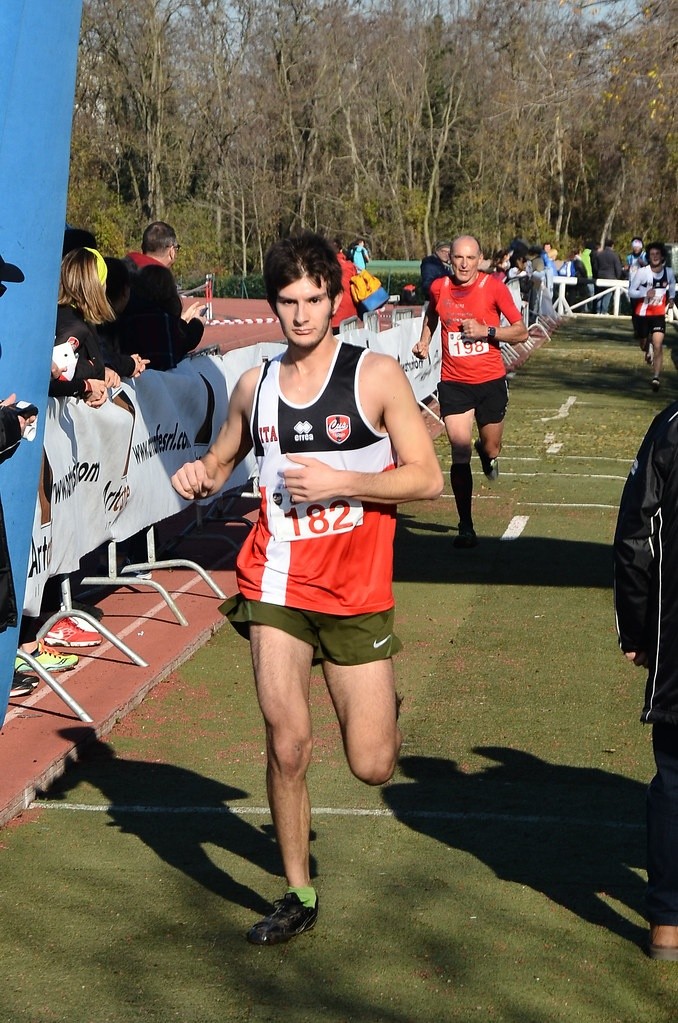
328 239 360 335
171 233 446 946
9 221 208 697
351 240 370 273
594 239 622 315
625 237 648 280
612 401 678 962
488 250 528 284
412 235 529 549
529 238 601 314
0 253 36 634
627 241 675 393
420 241 454 305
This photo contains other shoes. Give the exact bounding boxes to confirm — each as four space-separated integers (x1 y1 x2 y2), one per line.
649 919 678 962
116 558 153 580
9 674 40 696
645 344 655 365
651 378 660 392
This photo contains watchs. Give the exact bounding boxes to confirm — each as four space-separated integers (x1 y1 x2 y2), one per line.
488 327 495 338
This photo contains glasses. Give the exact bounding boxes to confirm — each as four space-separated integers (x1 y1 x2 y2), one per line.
166 243 180 253
439 249 450 255
0 284 7 297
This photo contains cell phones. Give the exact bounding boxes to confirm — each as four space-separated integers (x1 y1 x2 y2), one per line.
199 307 208 316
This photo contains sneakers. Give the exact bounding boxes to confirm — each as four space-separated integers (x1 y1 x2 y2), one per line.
473 438 499 481
246 889 320 946
69 600 104 622
13 643 79 673
43 615 103 646
454 526 476 552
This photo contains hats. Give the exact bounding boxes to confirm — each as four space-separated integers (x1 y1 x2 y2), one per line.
0 255 25 283
